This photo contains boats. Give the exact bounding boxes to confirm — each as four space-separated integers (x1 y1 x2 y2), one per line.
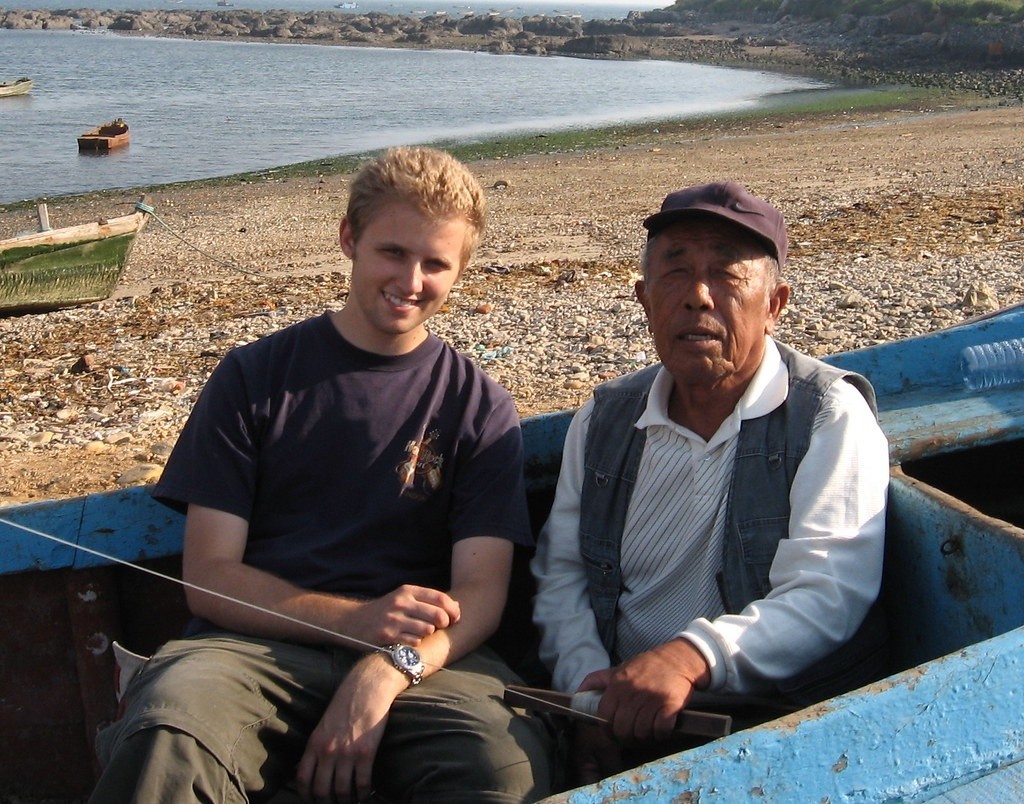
0 76 36 98
0 299 1024 804
75 115 132 150
0 192 159 318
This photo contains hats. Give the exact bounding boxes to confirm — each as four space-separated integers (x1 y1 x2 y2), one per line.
643 181 788 274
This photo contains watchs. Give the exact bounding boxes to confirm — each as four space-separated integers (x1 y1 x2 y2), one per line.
375 645 425 686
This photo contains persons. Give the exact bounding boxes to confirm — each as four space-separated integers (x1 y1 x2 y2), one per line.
89 143 551 804
529 180 891 779
